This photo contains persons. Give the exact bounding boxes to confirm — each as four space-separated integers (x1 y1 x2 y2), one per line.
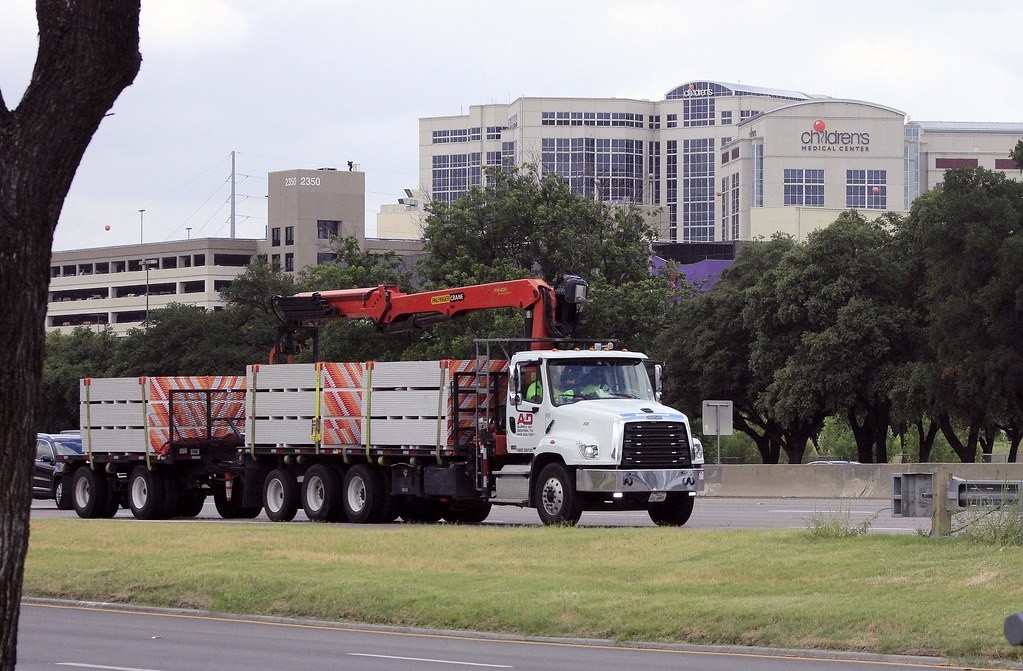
581 367 613 393
525 367 574 403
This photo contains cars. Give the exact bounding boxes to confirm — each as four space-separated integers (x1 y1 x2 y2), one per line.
32 431 87 510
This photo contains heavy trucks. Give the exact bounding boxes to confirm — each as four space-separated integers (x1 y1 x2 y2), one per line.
72 271 705 527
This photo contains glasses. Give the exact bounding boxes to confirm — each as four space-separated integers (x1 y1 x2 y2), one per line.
593 375 602 377
